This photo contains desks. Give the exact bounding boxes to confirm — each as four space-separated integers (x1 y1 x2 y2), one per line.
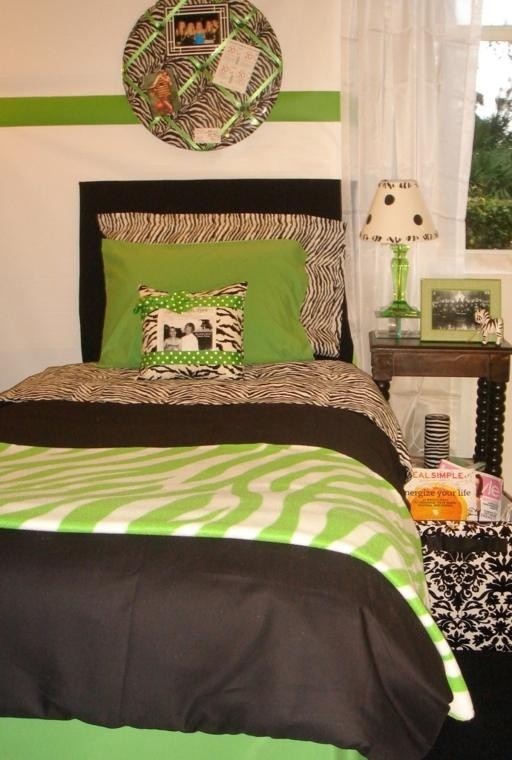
369 332 512 486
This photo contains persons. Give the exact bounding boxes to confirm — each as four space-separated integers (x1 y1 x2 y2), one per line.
475 474 483 522
432 296 489 316
180 324 199 351
176 20 220 46
164 327 182 351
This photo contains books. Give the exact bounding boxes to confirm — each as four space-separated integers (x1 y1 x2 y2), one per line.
405 457 508 527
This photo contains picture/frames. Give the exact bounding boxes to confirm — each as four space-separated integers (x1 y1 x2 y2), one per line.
414 277 504 342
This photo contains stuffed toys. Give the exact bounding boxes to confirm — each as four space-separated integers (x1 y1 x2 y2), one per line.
474 308 504 345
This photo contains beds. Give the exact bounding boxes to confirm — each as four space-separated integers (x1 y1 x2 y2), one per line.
2 173 483 758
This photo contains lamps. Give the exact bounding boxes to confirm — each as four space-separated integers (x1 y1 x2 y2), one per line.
358 176 441 338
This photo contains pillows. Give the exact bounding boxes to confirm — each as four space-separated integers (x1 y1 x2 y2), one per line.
135 282 250 383
95 240 315 368
98 214 348 358
